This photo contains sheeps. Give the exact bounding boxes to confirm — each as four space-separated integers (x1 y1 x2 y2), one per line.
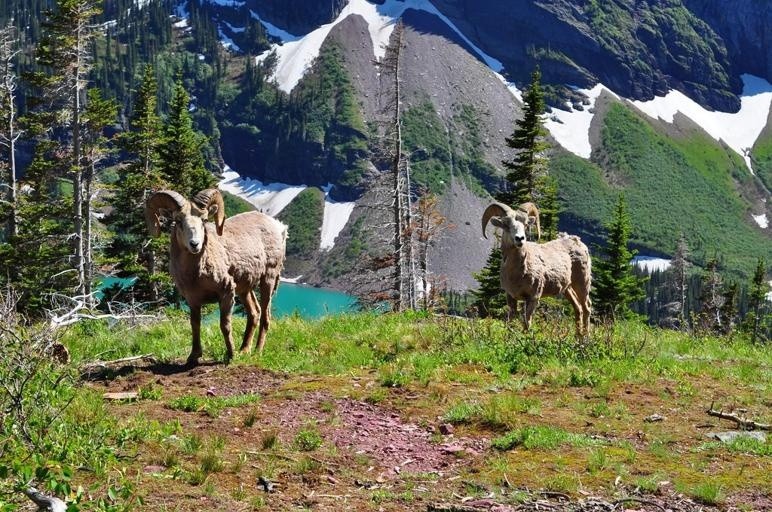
143 187 290 369
482 201 593 349
